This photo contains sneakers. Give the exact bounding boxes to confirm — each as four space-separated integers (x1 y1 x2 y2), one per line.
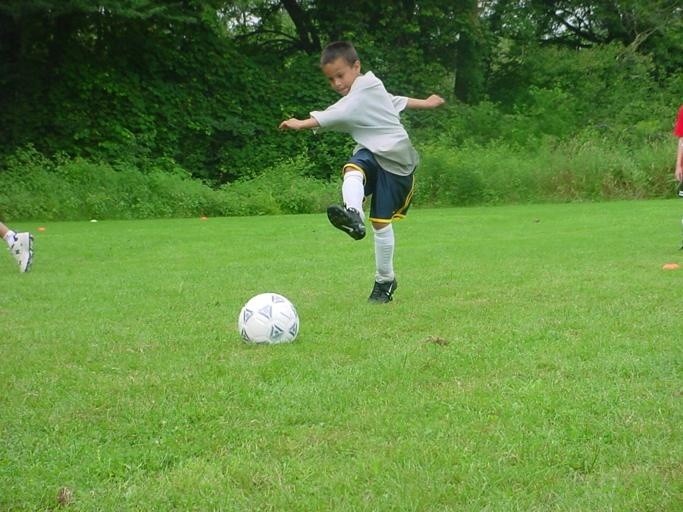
9 231 34 272
366 277 397 304
326 203 366 240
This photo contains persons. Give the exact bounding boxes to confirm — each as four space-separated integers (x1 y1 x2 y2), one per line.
278 39 445 303
672 104 683 183
0 222 34 274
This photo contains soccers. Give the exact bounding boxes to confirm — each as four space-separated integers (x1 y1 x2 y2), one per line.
238 293 300 345
